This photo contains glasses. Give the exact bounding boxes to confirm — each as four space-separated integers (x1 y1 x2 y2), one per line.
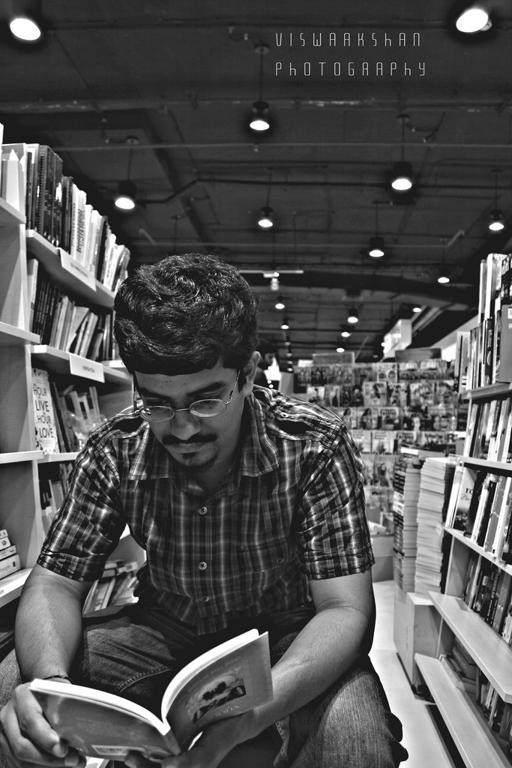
131 371 240 422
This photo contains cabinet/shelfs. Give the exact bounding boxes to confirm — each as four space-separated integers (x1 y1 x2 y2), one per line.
301 363 457 528
417 257 511 768
0 135 141 768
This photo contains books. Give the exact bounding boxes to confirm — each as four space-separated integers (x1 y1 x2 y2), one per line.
0 123 512 768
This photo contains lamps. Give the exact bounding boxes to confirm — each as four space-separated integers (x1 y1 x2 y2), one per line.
250 101 270 132
10 1 49 51
390 114 413 193
448 4 494 42
256 170 274 228
113 134 138 212
436 235 451 284
368 201 384 257
261 267 425 369
487 167 504 233
